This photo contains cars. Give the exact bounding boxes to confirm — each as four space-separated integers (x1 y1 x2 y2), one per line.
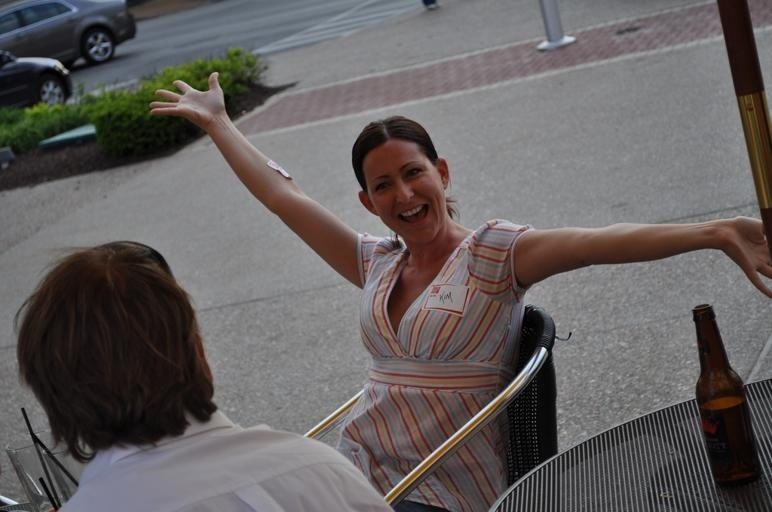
0 0 136 108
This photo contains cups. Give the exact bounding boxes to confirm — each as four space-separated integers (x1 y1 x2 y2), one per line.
4 432 84 512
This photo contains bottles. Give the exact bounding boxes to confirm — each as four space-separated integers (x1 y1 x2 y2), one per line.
691 303 763 485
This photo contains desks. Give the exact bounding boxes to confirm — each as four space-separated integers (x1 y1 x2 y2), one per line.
485 377 772 511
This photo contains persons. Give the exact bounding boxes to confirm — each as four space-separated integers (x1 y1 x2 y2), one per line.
12 237 399 512
146 68 772 511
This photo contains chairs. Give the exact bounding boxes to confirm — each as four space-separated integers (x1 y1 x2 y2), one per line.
301 304 559 512
